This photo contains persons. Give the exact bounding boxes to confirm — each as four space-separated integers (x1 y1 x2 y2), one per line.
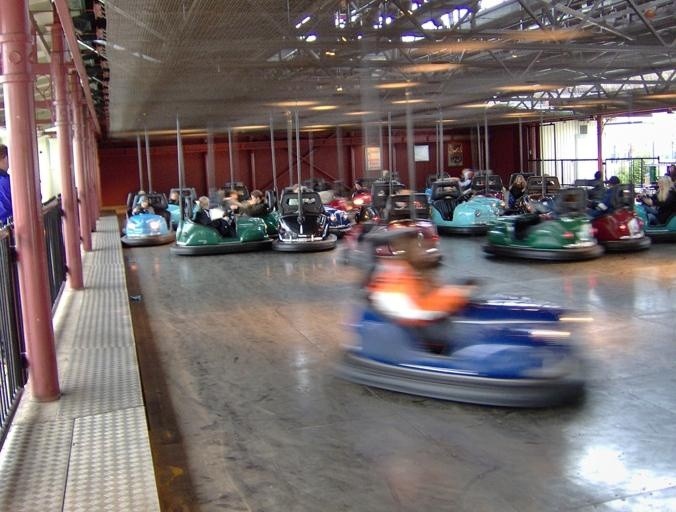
192 189 268 237
0 143 12 226
508 175 530 212
168 192 180 206
644 175 675 225
134 195 157 215
426 169 476 201
587 176 624 218
368 231 475 330
292 179 372 230
